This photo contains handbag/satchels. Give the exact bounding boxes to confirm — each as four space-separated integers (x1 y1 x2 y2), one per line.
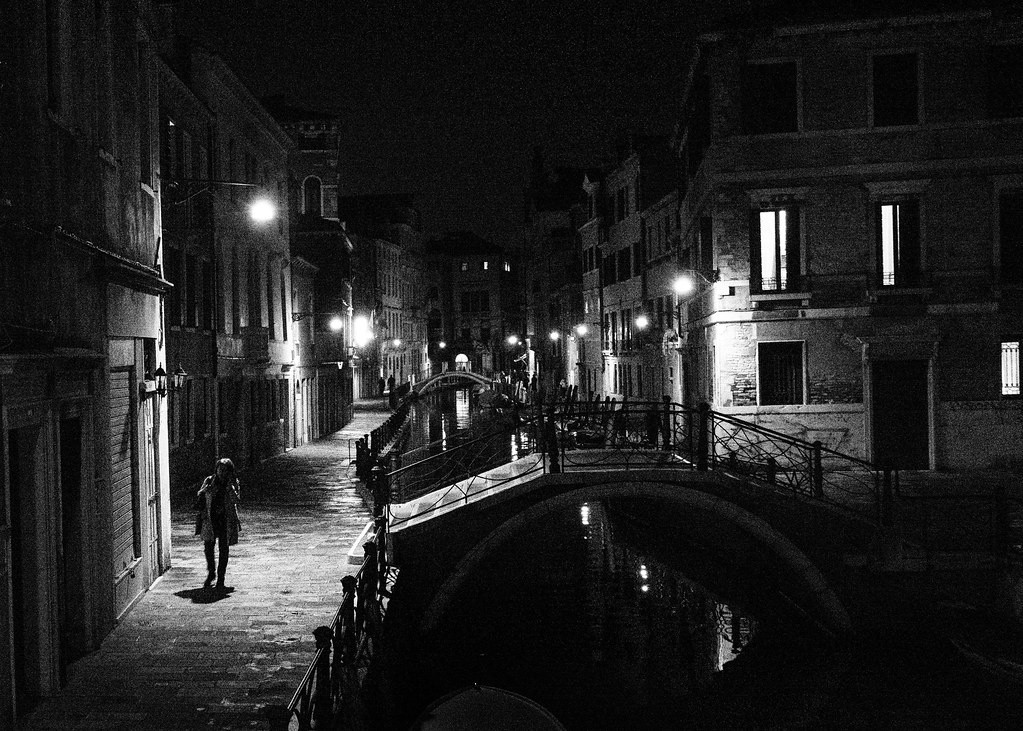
194 493 205 511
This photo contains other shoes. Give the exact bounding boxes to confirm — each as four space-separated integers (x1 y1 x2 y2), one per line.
215 584 235 594
204 572 215 588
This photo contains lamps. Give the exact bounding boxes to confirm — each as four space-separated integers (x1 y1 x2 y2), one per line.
342 338 358 366
161 360 187 402
141 362 168 403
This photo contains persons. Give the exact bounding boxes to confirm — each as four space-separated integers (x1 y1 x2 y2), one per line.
494 369 537 392
377 375 395 395
197 458 241 588
